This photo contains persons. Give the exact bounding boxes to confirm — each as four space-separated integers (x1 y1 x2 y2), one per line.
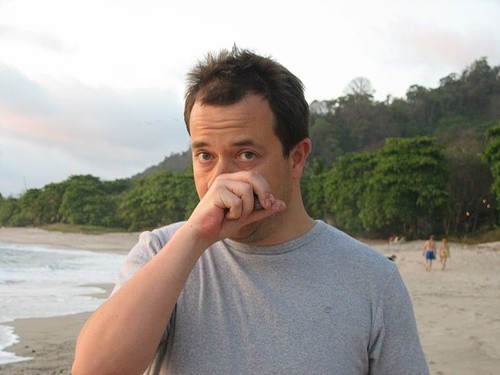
439 238 451 271
422 235 437 273
72 41 430 375
